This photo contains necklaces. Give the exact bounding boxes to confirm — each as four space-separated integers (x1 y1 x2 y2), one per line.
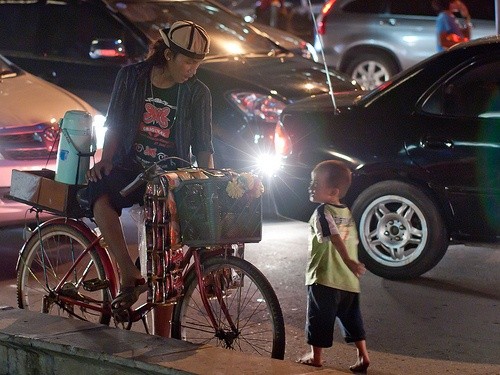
151 65 180 131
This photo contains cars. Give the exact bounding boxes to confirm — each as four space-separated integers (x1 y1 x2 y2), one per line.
271 35 499 280
1 0 499 205
1 52 107 229
15 156 288 361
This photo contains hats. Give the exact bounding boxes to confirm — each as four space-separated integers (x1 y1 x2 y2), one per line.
159 20 209 58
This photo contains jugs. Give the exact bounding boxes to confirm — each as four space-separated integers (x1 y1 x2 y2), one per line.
55 110 97 184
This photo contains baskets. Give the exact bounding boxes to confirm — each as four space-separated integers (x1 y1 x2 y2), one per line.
172 178 262 247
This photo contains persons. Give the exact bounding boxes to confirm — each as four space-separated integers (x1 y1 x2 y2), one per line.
83 20 216 337
295 159 370 371
433 0 471 51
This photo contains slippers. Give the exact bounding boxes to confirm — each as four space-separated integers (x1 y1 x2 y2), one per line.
111 284 148 313
